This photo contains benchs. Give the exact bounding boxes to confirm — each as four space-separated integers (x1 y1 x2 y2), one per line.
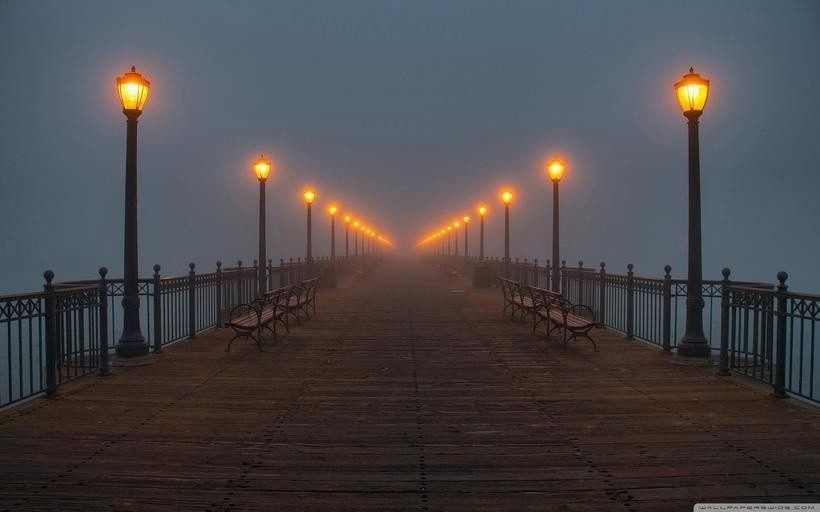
496 275 602 353
223 275 321 353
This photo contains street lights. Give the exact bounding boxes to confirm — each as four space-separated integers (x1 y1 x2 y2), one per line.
344 216 396 263
327 206 339 264
110 64 151 361
544 157 565 292
301 190 317 269
414 216 471 271
478 203 489 261
252 150 272 306
500 189 511 278
672 64 714 360
